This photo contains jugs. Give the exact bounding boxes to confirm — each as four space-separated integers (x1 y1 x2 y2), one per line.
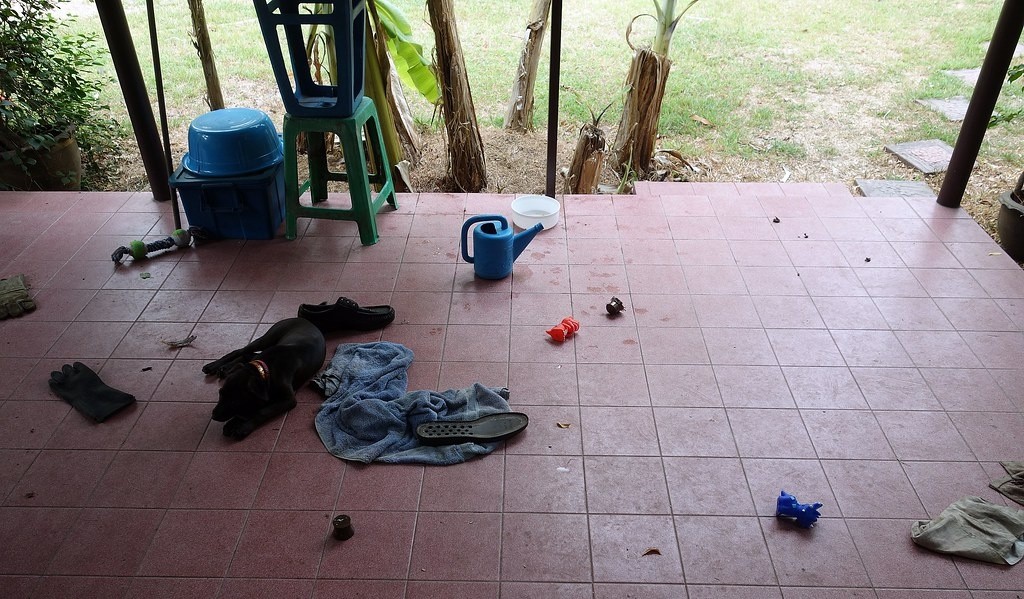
460 214 544 279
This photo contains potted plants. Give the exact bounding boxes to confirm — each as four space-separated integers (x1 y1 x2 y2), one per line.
0 0 129 191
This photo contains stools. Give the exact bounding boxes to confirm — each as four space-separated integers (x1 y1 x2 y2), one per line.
281 96 399 246
251 0 367 118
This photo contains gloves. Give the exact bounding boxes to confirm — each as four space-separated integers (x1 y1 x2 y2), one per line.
48 362 136 424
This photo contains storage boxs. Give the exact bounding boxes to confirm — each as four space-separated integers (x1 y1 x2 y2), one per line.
167 133 286 244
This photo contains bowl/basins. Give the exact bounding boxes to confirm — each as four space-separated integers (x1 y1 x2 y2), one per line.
179 107 284 176
511 195 561 231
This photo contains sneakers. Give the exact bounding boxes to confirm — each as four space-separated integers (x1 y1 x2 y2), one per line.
298 297 396 334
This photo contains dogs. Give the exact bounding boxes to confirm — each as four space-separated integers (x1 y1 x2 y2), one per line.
201 301 328 442
